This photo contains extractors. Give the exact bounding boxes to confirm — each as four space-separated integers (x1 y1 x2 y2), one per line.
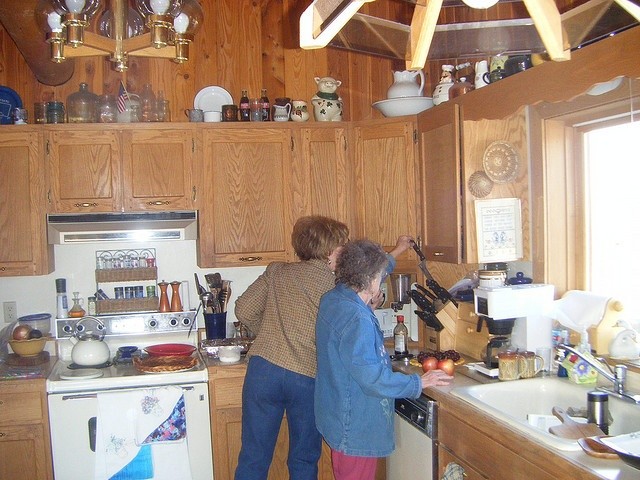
46 210 197 244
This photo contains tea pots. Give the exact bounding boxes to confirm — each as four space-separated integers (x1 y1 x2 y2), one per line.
482 66 505 85
481 337 514 367
69 314 110 365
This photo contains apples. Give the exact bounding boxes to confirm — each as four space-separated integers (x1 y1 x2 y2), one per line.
423 357 438 372
438 358 455 375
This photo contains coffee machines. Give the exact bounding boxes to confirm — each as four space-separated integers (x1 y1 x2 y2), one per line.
471 271 555 378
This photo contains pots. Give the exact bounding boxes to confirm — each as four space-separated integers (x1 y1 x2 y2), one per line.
16 313 52 336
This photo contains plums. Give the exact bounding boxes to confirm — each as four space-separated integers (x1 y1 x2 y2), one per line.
31 330 42 338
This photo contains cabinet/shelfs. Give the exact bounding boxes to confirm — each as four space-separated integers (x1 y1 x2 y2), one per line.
435 403 600 480
196 123 290 267
208 365 386 479
419 105 532 263
44 123 196 212
291 112 423 269
0 125 54 277
1 379 53 480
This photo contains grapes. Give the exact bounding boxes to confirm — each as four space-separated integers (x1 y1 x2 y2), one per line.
418 350 461 363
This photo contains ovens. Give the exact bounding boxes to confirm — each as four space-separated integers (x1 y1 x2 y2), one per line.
46 383 216 480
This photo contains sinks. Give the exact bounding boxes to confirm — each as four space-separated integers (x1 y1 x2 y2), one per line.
453 376 640 449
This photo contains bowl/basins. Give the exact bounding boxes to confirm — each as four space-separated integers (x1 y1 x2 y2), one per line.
143 344 197 357
8 336 48 358
371 97 435 117
596 434 640 471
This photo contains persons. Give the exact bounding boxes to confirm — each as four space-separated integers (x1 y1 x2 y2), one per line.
233 214 345 480
315 237 454 480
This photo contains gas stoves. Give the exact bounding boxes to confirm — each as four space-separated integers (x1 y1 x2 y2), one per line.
45 351 206 393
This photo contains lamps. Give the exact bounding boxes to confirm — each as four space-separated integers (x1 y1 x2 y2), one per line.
45 0 203 71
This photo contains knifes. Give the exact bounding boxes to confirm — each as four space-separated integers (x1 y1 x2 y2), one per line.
404 278 457 352
409 240 434 281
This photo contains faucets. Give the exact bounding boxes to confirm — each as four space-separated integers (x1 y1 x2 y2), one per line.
558 343 627 394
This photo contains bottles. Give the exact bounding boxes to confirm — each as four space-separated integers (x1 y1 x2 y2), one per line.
393 315 408 356
130 257 139 268
97 94 116 123
139 257 146 268
96 257 104 270
114 287 124 299
66 82 97 124
169 281 182 312
251 97 260 121
158 280 171 312
88 297 96 315
259 88 270 120
147 259 154 267
94 289 110 300
588 391 609 435
123 255 131 269
34 102 47 124
291 101 310 122
113 258 121 268
137 84 157 122
68 291 86 318
147 286 155 297
272 98 292 120
125 287 133 299
158 100 171 122
105 259 112 269
55 278 69 318
135 286 143 298
47 102 65 123
118 100 142 122
239 90 250 121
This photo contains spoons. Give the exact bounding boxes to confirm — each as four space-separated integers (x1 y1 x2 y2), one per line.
199 292 213 312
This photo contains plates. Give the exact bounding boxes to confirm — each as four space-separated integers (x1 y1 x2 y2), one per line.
0 86 23 124
194 85 233 122
469 172 494 197
578 438 620 459
482 139 522 185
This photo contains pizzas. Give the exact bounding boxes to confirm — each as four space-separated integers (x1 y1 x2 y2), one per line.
139 355 197 372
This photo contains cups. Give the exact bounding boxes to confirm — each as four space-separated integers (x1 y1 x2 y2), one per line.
497 350 527 381
203 312 227 339
475 60 488 90
531 53 547 67
490 55 508 72
205 111 222 123
222 105 238 122
184 109 204 122
518 351 544 378
272 103 291 122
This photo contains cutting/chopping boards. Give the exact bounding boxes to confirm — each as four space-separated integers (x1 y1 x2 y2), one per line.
549 405 606 439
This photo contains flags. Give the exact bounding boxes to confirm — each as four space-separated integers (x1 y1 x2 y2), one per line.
113 83 127 115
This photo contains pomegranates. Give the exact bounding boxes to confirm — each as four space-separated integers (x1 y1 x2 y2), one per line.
13 326 31 340
